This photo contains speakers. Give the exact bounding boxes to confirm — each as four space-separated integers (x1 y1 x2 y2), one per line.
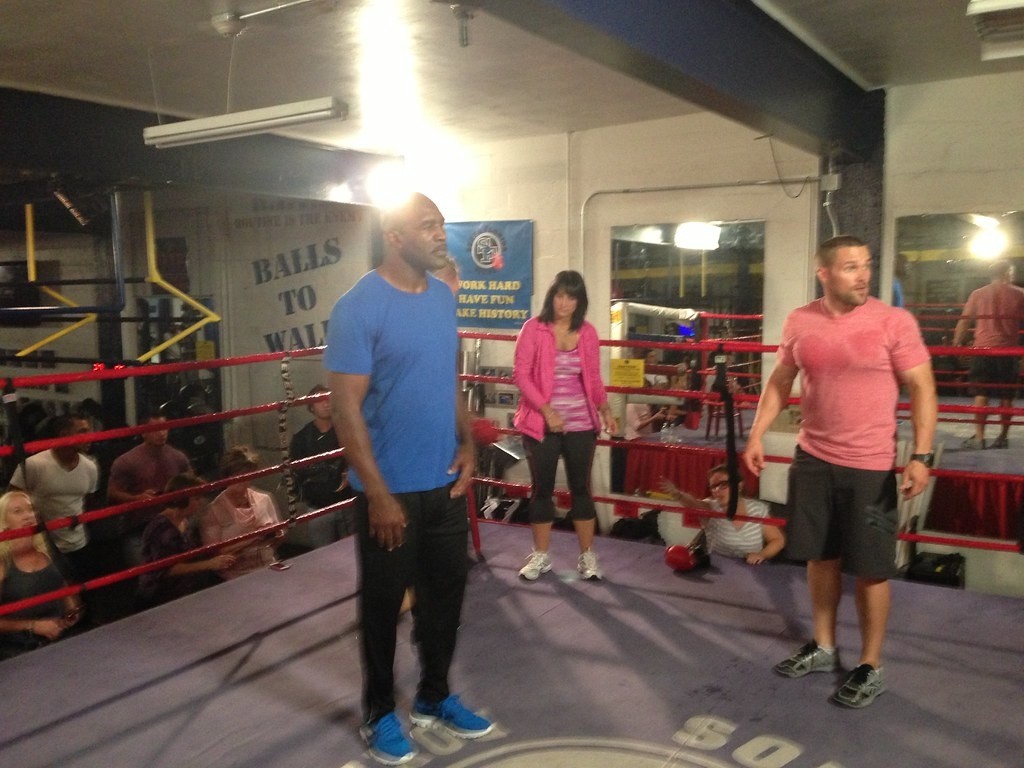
906 551 965 591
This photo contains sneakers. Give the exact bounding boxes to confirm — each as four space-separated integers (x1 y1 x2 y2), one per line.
408 693 495 739
575 547 604 582
517 547 553 580
359 711 416 766
830 662 889 708
774 637 840 678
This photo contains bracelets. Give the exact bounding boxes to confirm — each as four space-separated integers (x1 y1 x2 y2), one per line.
601 408 610 415
28 618 33 635
673 491 684 500
546 412 555 422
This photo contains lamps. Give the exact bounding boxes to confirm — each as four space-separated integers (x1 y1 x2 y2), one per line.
143 97 350 153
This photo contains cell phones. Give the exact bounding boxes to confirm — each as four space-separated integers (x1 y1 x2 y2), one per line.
61 605 85 621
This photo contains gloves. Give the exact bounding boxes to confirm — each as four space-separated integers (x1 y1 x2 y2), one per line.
665 544 711 572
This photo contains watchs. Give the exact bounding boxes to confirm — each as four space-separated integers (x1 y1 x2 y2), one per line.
909 451 935 467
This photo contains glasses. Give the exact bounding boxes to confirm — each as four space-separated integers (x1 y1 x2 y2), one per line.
709 479 729 491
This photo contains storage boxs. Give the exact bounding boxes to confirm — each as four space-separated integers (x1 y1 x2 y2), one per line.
910 552 967 592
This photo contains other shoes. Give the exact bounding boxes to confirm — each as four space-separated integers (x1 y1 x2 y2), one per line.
960 434 985 450
990 436 1008 449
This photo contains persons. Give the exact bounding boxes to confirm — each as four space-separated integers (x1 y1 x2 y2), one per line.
107 411 208 590
198 444 290 580
0 487 82 655
5 413 100 627
742 236 937 708
513 270 618 581
622 327 739 446
323 189 500 759
163 319 196 394
394 254 470 647
284 386 363 549
950 257 1023 450
0 384 224 513
656 466 786 564
890 250 910 307
137 472 237 604
